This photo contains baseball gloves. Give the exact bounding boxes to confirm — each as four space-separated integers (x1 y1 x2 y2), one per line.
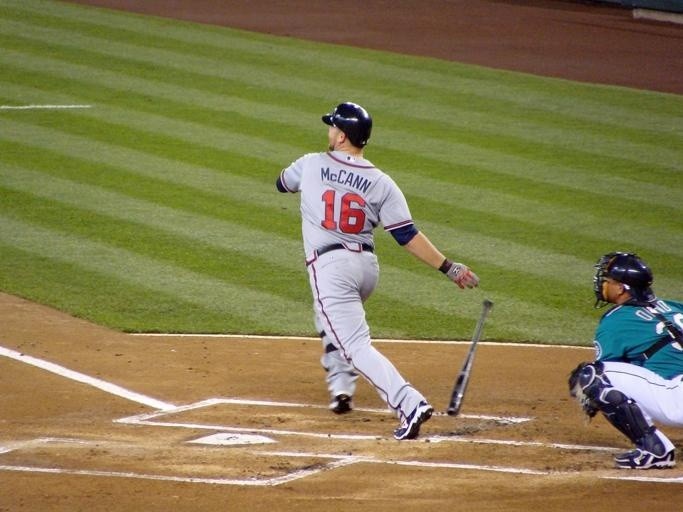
569 361 599 417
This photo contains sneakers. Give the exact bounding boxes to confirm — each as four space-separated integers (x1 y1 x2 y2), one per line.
328 395 355 412
393 400 434 440
612 447 676 470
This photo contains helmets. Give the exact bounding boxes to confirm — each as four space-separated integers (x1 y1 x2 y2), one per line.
322 101 373 144
592 250 657 309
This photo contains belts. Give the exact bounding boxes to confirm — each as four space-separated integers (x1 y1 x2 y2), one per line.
316 241 374 255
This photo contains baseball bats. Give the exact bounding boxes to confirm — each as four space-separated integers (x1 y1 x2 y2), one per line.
446 298 493 415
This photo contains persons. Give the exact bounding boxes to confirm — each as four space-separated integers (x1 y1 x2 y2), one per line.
275 103 480 441
567 251 682 472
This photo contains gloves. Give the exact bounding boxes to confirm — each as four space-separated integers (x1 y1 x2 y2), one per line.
439 258 481 290
